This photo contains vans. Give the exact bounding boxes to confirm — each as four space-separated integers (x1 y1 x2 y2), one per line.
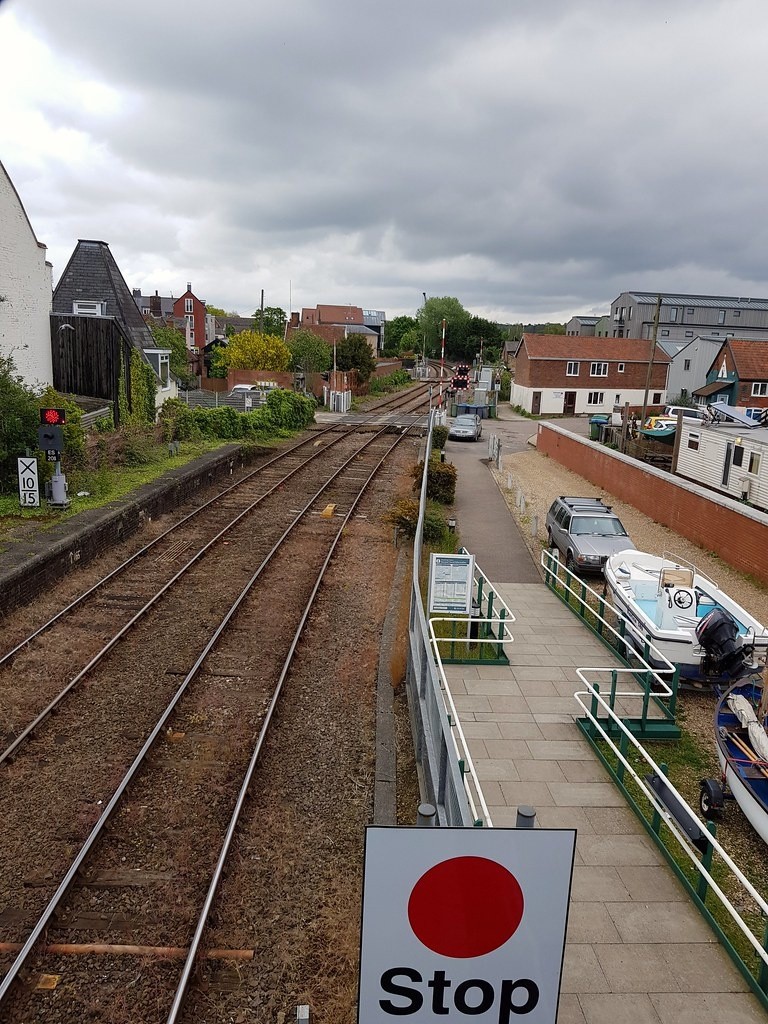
663 405 714 425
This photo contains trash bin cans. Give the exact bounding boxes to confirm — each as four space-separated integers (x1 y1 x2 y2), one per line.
450 403 495 419
588 414 611 441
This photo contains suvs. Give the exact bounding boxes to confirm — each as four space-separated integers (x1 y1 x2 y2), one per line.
545 494 639 578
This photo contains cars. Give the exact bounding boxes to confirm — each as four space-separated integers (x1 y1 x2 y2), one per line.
654 421 677 430
448 413 482 442
644 416 677 430
228 384 284 398
664 426 677 430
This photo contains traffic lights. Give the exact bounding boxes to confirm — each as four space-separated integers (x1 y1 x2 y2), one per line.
39 408 66 425
39 427 64 451
321 372 330 382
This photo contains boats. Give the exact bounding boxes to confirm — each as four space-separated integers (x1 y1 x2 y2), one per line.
603 547 768 683
714 675 768 848
